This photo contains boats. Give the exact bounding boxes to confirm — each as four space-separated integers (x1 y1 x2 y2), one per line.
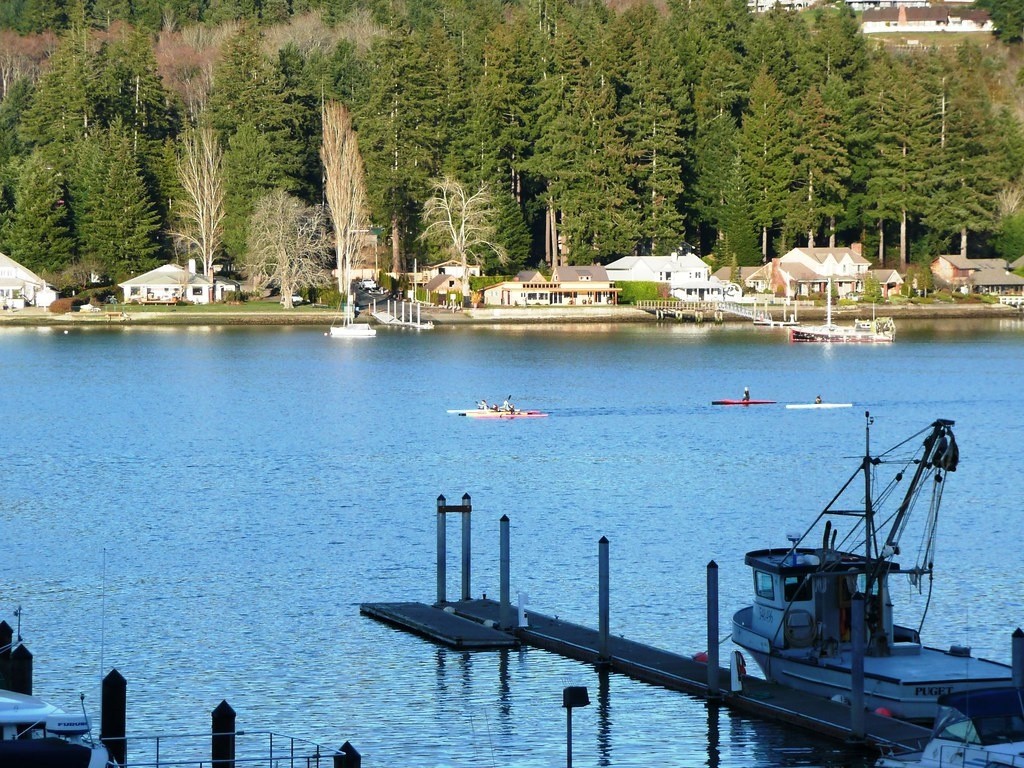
731 409 1018 725
787 300 897 344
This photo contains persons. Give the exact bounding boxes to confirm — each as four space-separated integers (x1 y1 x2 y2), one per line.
760 312 764 322
814 396 822 404
742 386 750 401
790 313 794 322
478 400 514 414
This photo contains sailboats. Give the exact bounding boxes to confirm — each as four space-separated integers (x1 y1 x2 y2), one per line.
329 254 377 336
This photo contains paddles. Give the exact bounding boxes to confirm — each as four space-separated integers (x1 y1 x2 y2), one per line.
507 395 511 402
474 400 493 409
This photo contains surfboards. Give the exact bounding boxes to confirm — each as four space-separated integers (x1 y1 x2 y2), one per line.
712 399 777 404
785 403 852 408
